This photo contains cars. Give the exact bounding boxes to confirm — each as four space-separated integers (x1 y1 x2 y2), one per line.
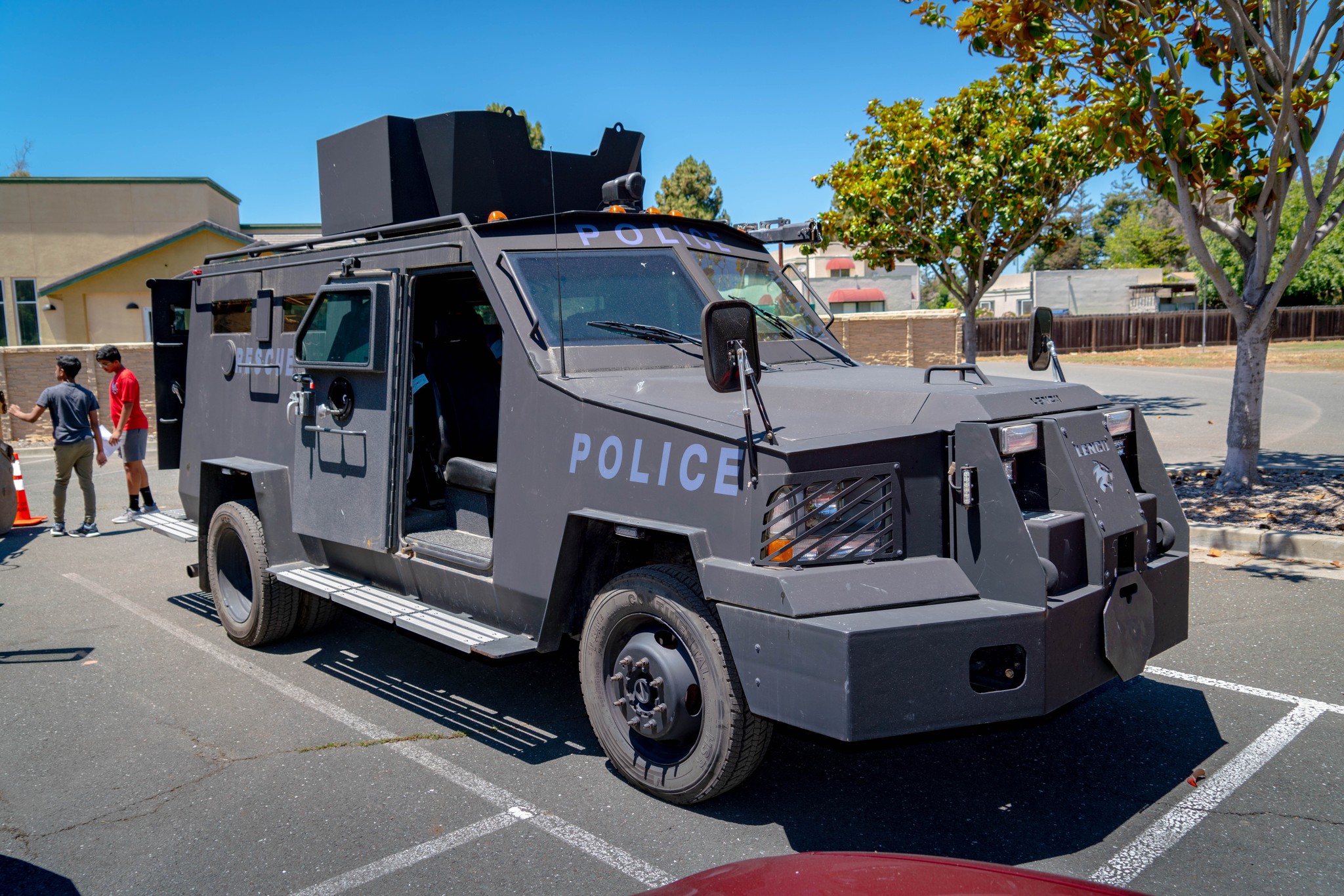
625 851 1158 896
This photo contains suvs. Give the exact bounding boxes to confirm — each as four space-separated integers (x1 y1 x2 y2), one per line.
145 106 1188 808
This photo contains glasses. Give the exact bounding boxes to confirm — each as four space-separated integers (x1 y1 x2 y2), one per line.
98 361 111 368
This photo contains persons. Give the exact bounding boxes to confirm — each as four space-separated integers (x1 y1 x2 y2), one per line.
96 345 160 523
8 354 108 537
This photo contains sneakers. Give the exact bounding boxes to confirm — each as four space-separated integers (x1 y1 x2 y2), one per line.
67 522 101 537
140 503 161 515
50 522 66 536
111 506 141 523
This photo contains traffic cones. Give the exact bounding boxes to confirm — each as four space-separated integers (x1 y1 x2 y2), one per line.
11 452 47 527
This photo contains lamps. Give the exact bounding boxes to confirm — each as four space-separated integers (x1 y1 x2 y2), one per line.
126 303 139 309
42 304 56 310
